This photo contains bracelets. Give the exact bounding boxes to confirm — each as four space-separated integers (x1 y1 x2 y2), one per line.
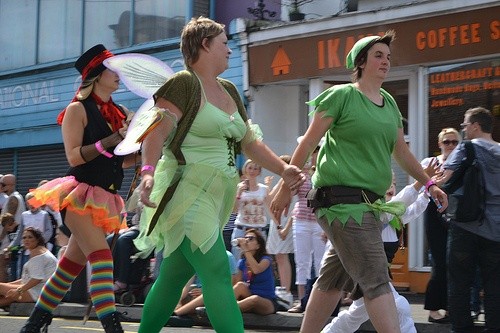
17 286 25 294
96 140 113 158
277 225 281 229
426 180 437 192
141 165 154 172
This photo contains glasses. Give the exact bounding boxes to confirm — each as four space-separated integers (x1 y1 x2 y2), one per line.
443 140 458 146
242 236 256 241
0 183 13 187
460 123 472 128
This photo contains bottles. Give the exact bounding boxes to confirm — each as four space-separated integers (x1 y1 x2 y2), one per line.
275 286 286 295
231 238 241 247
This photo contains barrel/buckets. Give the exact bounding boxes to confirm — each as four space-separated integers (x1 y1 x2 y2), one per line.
408 266 433 293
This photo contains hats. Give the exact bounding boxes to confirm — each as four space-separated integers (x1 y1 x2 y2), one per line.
75 44 117 82
346 36 381 70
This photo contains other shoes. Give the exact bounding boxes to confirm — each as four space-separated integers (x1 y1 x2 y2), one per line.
341 297 353 306
288 303 303 312
196 306 208 318
428 315 450 324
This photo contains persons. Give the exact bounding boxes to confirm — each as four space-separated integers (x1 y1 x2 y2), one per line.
0 227 59 308
271 32 448 333
233 229 278 315
166 250 238 328
0 173 63 279
137 16 307 333
320 157 445 333
288 145 328 312
106 199 145 293
230 158 268 259
440 107 500 333
421 128 481 322
55 224 72 259
20 44 134 333
265 155 294 309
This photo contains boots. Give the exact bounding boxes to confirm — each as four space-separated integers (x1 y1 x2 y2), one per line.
20 306 53 333
99 310 124 333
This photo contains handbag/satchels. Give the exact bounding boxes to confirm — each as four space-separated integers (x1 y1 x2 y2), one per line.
442 141 486 222
